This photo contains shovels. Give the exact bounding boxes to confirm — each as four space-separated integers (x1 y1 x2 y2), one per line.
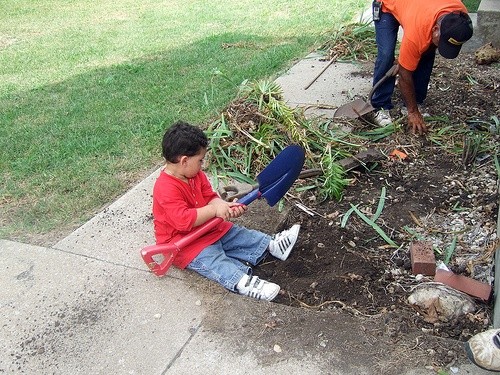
332 65 398 122
141 144 306 277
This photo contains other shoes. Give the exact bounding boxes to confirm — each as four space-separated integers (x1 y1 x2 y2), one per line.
465 329 500 372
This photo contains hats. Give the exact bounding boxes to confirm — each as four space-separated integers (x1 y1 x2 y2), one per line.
437 10 474 60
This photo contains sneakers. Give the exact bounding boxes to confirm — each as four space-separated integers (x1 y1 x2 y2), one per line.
402 106 430 119
269 224 301 261
373 108 392 128
236 274 280 302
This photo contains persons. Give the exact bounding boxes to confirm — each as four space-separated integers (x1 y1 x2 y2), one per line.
366 0 473 138
465 328 500 372
152 120 300 302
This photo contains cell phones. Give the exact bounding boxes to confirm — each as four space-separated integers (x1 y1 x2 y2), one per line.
373 2 382 21
